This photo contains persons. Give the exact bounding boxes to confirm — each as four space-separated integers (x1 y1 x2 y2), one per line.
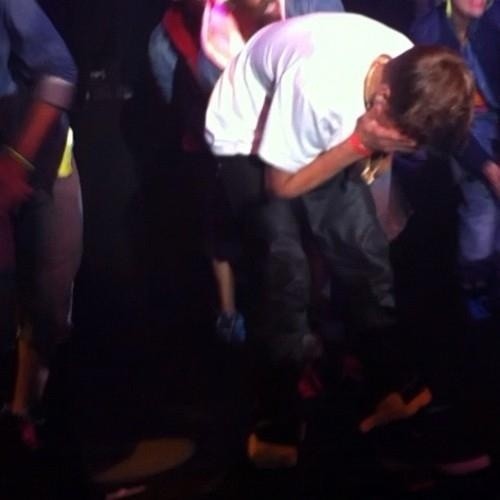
2 0 499 466
